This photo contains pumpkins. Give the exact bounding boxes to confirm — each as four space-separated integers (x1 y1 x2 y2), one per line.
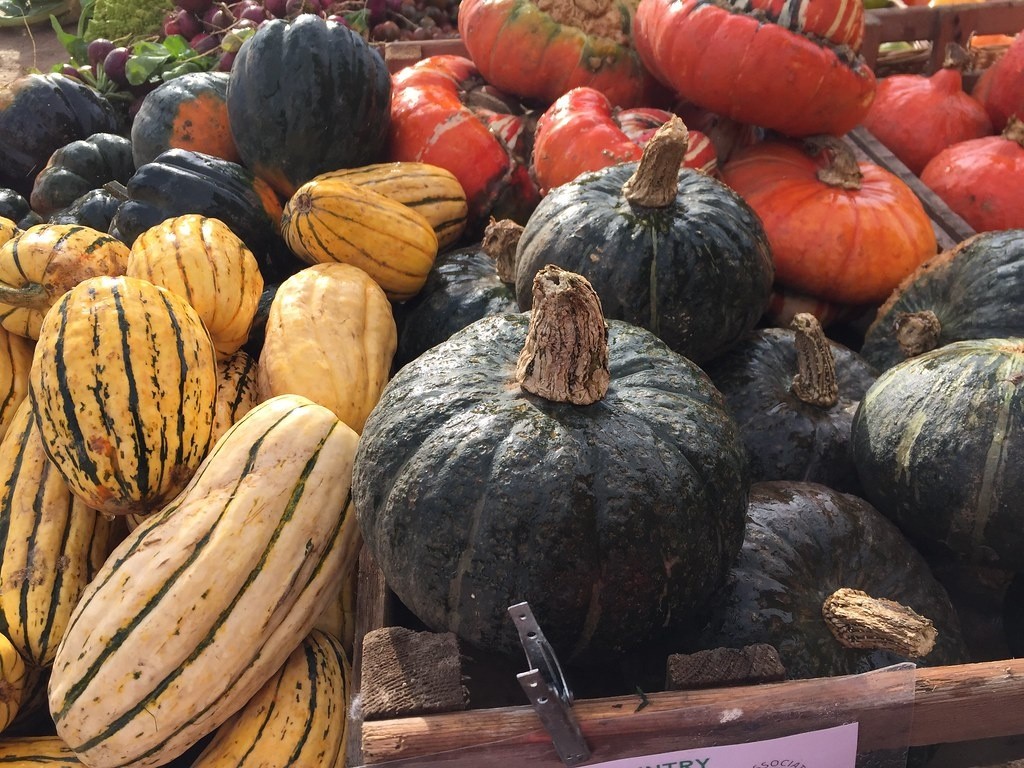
0 0 1024 768
